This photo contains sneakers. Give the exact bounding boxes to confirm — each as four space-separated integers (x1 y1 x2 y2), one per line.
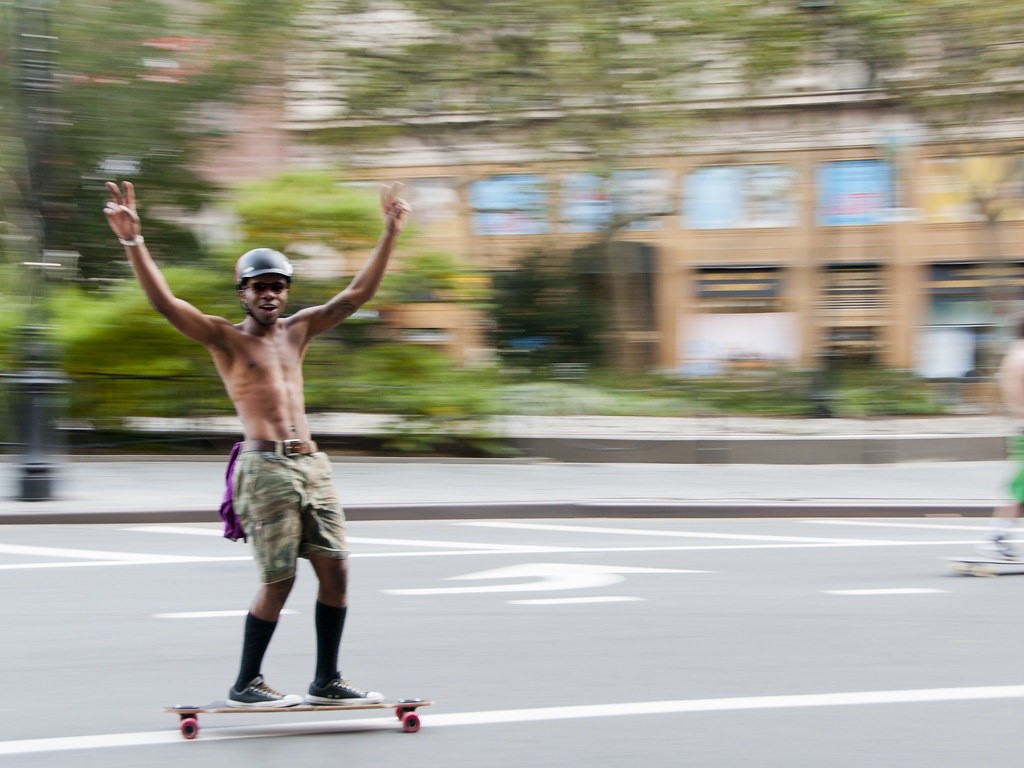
305 670 383 705
227 674 303 708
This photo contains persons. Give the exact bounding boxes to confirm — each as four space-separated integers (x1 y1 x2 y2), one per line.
104 181 412 710
990 313 1024 558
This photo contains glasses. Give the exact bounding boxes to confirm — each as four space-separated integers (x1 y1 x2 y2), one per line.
247 281 283 292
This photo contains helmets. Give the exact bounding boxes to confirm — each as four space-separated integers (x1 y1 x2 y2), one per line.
234 248 293 285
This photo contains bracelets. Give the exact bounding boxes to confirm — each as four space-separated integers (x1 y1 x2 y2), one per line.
119 235 144 246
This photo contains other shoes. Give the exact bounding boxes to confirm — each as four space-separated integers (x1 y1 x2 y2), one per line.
993 534 1013 556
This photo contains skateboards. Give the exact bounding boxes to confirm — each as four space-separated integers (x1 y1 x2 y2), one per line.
941 556 1024 577
159 697 436 740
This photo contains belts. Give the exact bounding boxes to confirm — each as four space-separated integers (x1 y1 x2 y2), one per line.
239 439 318 457
